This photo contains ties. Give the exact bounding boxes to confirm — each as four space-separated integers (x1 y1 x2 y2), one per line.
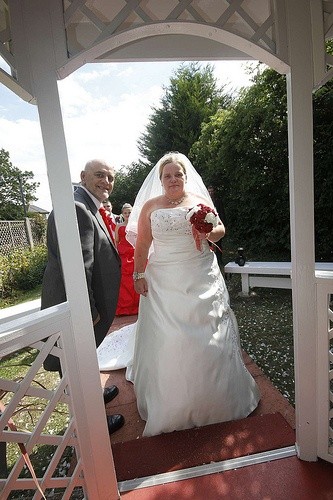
100 208 115 247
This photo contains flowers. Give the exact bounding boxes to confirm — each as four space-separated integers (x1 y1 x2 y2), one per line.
115 217 121 223
186 203 218 251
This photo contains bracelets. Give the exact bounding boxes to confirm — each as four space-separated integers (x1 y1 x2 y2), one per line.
133 272 145 281
205 233 210 240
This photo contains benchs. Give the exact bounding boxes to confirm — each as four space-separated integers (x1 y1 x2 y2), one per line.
0 298 41 326
224 261 333 293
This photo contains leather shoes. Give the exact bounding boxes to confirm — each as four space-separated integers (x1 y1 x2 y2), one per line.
103 385 119 403
107 414 125 435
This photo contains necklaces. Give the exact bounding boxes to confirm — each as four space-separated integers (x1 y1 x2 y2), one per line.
165 194 181 208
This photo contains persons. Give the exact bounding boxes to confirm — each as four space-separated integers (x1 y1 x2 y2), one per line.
39 160 125 434
132 156 225 393
114 204 147 306
98 199 125 240
